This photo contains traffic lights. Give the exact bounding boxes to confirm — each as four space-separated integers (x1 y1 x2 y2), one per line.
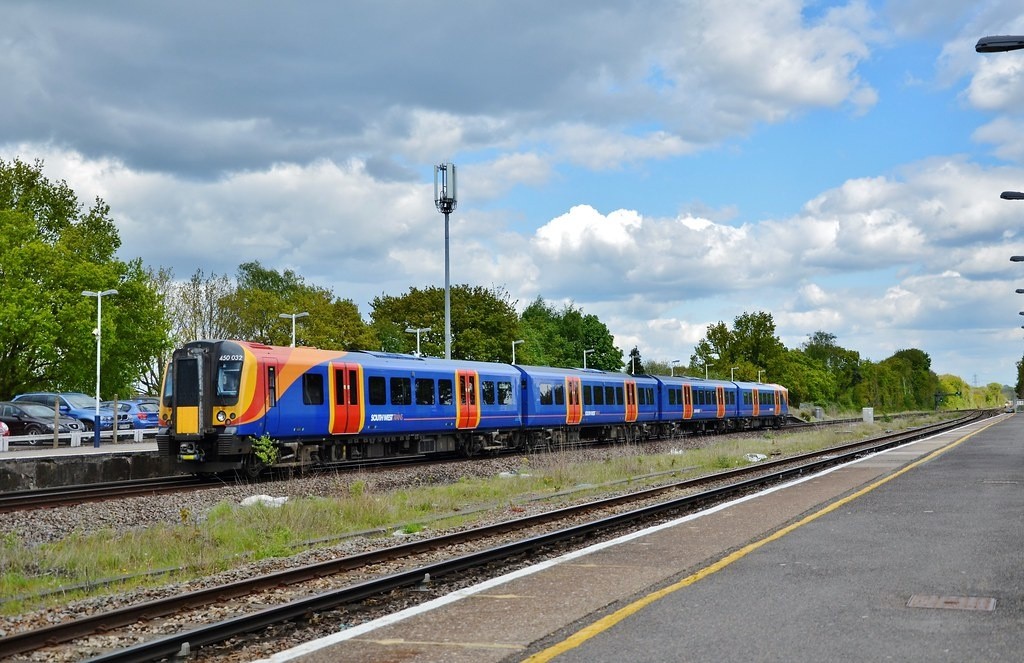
940 397 943 402
955 391 961 395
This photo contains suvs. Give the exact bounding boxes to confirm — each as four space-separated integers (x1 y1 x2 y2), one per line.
9 392 135 441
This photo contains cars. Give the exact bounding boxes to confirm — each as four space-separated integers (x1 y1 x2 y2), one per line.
100 396 160 439
0 400 85 445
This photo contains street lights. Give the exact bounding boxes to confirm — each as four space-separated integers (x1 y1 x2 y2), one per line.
671 360 679 376
278 312 310 346
583 349 595 369
512 340 525 365
80 289 119 448
758 370 765 383
432 162 459 359
405 328 432 357
631 355 642 375
705 363 715 379
730 366 739 382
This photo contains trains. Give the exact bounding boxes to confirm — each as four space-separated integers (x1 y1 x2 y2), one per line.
156 339 790 479
